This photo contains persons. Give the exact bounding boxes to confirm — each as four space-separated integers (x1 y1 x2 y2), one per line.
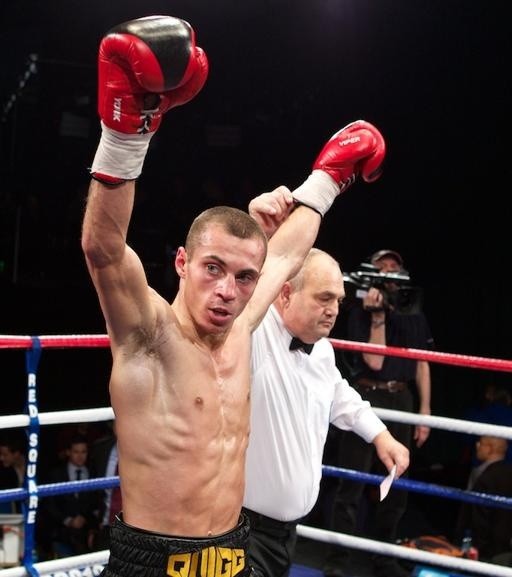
341 248 432 537
242 183 413 577
79 14 389 576
451 435 509 562
1 430 123 559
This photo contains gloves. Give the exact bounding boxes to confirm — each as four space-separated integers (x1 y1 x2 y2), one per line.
90 14 212 188
289 118 388 217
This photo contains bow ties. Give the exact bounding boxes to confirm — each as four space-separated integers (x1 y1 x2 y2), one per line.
289 334 316 355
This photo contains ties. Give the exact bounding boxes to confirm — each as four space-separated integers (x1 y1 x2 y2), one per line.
73 469 83 482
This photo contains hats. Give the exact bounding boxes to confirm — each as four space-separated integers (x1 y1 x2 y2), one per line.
371 246 404 266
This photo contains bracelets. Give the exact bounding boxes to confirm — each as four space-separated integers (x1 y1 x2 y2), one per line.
370 319 386 326
